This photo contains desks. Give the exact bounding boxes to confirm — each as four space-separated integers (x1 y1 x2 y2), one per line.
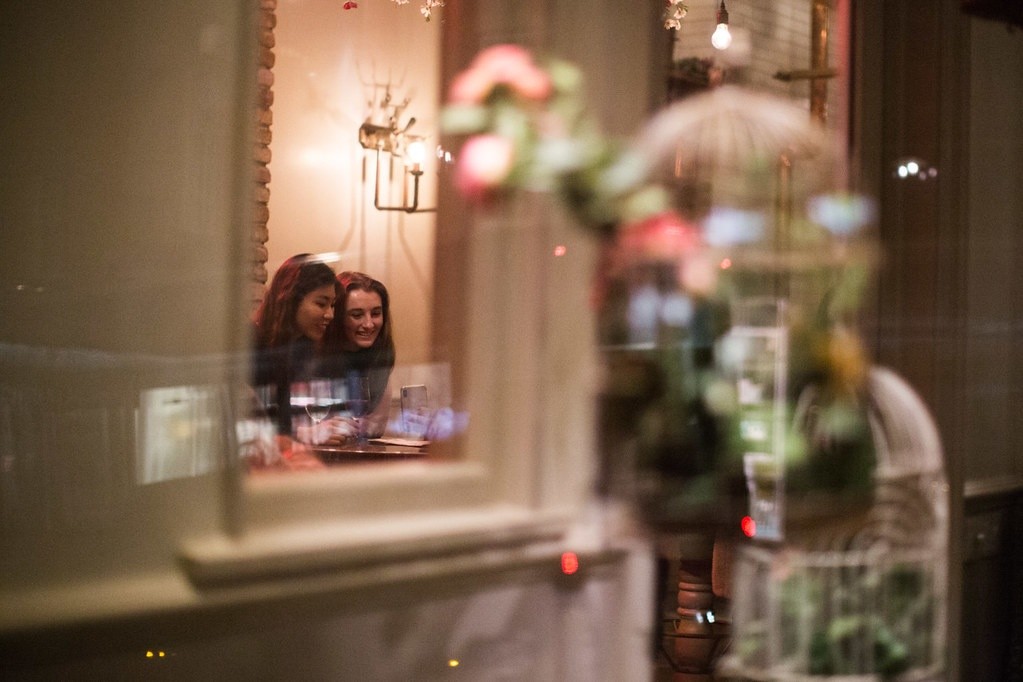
313 436 426 466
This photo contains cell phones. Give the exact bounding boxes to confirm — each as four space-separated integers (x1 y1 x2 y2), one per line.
400 383 429 435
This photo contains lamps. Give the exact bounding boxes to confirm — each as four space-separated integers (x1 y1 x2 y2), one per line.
359 117 426 215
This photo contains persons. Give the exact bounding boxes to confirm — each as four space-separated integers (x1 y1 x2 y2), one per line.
254 253 361 444
291 270 395 445
